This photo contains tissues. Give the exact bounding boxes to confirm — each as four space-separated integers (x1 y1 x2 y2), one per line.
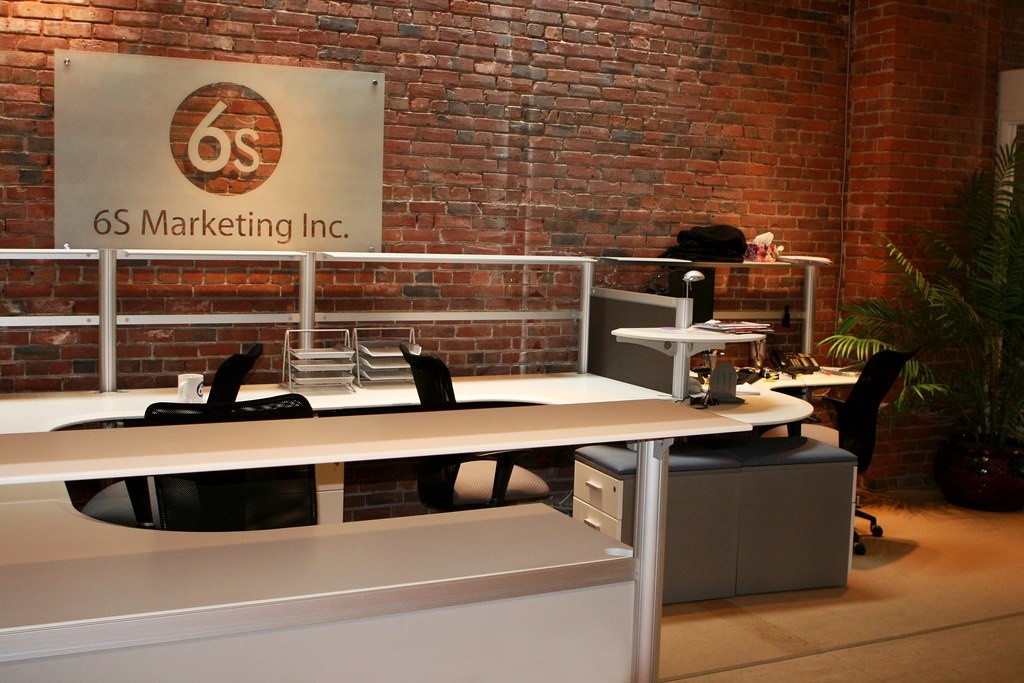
744 232 776 262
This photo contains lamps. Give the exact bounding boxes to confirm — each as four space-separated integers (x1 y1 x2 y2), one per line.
683 269 704 298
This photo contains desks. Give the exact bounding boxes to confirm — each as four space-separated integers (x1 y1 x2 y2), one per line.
0 249 863 683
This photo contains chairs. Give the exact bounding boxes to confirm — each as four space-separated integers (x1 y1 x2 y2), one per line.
398 342 550 512
82 394 316 532
759 347 924 554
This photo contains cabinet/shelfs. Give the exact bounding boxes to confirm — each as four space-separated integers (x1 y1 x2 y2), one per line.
572 437 861 605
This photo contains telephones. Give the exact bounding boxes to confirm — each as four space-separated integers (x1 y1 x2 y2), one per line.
768 346 821 372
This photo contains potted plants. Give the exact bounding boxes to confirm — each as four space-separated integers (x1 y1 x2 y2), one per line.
816 133 1024 511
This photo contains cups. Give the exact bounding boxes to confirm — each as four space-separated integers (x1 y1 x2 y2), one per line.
178 374 204 403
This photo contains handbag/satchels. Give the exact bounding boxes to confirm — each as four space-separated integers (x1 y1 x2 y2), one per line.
657 224 748 262
